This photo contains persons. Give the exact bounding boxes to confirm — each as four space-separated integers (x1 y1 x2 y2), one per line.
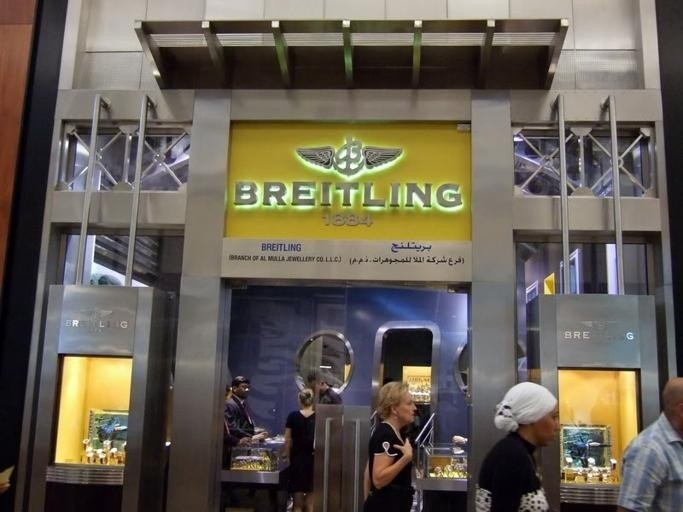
224 376 268 441
221 385 249 470
307 370 342 404
363 381 417 512
283 389 315 512
616 377 682 512
473 382 560 512
